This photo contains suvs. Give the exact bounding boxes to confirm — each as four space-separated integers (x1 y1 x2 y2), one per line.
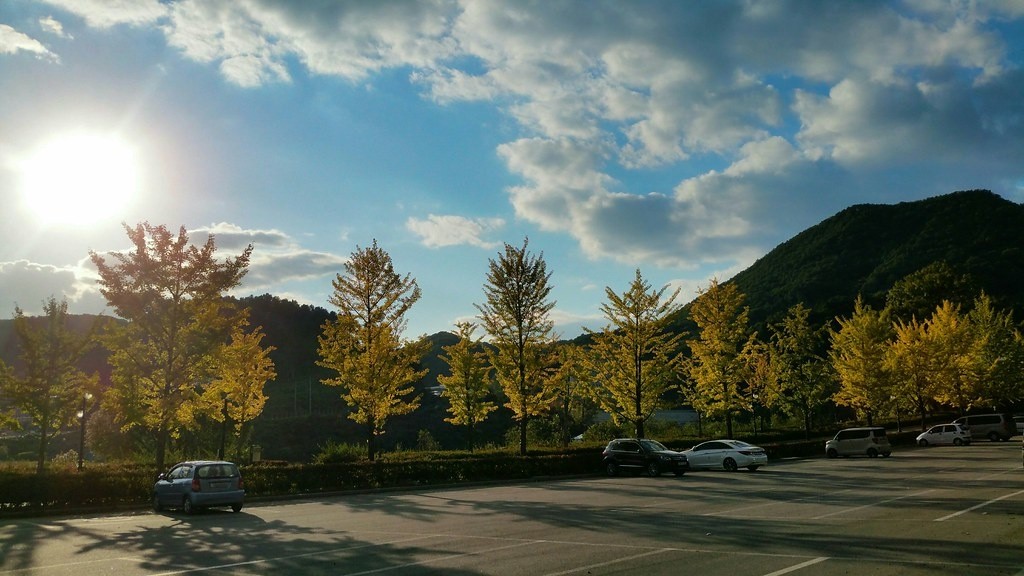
602 438 690 478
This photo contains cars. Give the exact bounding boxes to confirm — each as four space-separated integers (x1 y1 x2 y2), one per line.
916 423 973 448
1013 416 1024 435
153 460 246 516
680 439 768 474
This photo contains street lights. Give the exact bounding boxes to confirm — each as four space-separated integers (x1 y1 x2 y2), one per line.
76 390 93 472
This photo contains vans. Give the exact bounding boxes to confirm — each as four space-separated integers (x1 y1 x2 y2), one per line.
825 427 892 459
951 413 1017 442
